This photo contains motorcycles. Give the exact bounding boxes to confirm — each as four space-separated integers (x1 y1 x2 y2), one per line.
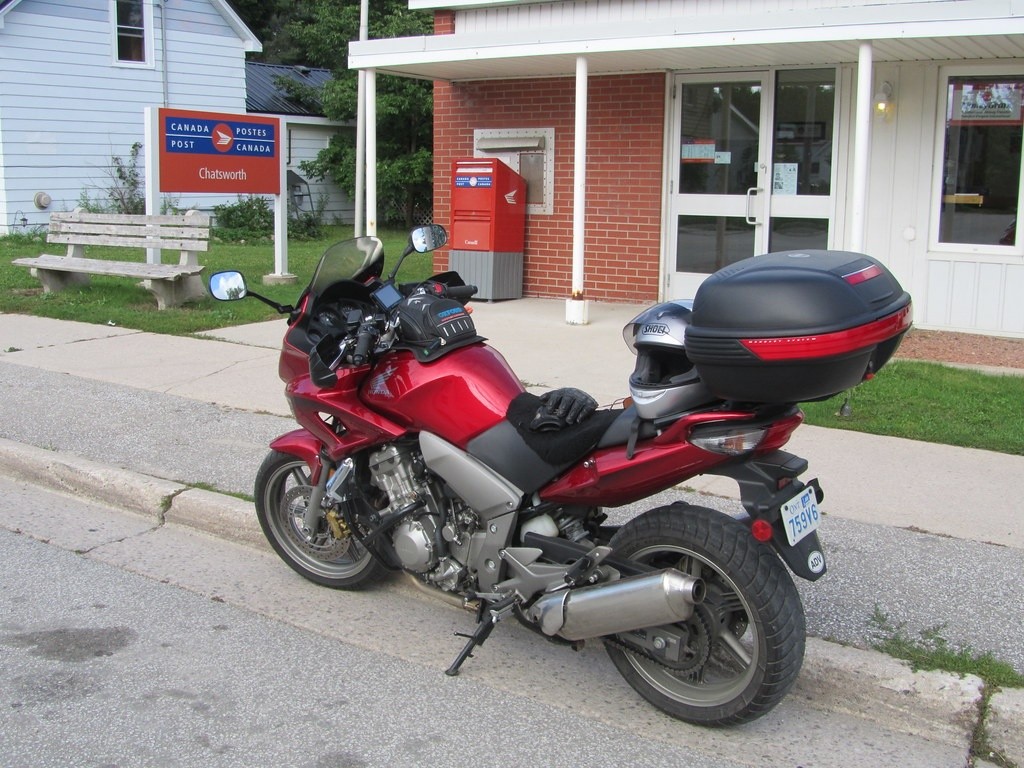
207 222 914 732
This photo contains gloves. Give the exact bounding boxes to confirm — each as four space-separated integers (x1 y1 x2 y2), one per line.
530 387 599 432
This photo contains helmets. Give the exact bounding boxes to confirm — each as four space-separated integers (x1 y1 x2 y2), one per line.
629 300 725 419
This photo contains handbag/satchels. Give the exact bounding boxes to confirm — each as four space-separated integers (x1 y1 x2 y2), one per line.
396 294 477 356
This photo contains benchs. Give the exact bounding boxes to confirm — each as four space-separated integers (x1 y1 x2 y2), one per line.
11 207 212 312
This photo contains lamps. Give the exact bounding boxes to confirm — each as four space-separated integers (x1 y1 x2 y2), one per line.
872 80 893 110
